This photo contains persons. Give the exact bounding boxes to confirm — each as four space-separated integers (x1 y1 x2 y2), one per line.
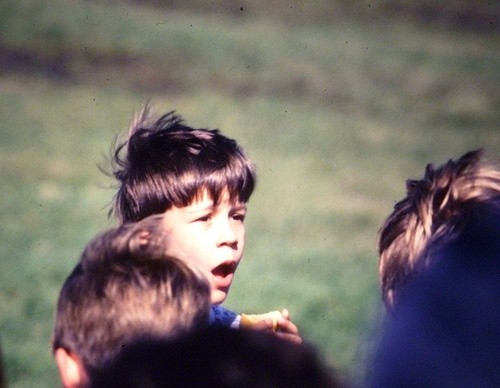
51 214 212 388
378 146 500 315
81 324 354 388
96 97 303 345
